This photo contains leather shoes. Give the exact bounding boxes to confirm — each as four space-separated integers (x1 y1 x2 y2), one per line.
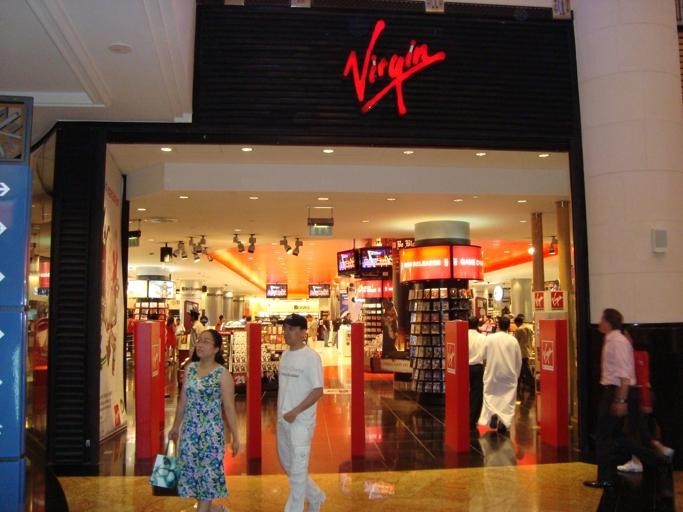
582 480 611 488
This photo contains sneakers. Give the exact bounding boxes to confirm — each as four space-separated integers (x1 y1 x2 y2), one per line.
617 461 643 474
308 491 326 512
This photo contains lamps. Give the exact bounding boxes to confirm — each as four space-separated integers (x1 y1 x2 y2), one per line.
189 235 212 265
277 232 305 257
545 233 559 256
231 232 260 254
172 239 189 261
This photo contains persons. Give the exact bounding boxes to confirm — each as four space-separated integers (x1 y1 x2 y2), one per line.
126 309 352 361
468 313 534 432
168 329 239 512
583 309 670 488
275 314 326 512
617 326 674 473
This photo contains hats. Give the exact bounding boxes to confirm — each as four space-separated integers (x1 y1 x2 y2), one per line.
276 313 307 327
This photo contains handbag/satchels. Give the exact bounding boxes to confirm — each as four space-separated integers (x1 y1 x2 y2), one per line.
148 438 180 498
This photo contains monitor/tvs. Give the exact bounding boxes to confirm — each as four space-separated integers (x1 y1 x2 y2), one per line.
337 248 359 275
359 246 392 278
266 284 288 298
309 284 331 298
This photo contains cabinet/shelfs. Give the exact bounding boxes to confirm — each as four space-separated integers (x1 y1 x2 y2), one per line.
334 283 535 403
127 278 306 395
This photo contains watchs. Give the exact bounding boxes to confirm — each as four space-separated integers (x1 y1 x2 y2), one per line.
614 398 627 404
